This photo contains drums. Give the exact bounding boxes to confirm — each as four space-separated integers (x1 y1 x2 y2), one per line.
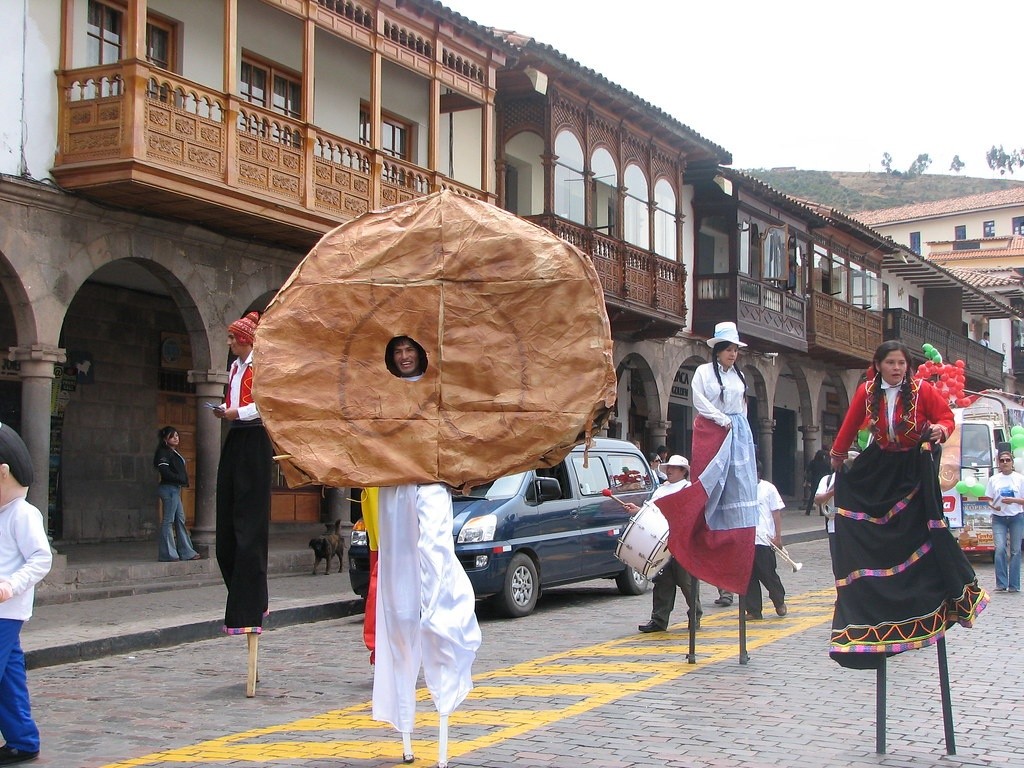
615 501 673 580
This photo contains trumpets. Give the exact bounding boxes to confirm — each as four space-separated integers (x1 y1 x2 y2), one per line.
756 531 804 573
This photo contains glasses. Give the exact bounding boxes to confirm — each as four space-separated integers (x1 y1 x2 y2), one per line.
1000 459 1011 463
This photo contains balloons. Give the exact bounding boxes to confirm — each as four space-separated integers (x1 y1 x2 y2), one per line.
857 427 871 450
915 343 986 408
1009 425 1024 475
957 478 986 497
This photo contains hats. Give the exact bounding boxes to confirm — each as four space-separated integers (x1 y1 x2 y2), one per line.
658 455 691 481
228 312 260 345
707 322 748 349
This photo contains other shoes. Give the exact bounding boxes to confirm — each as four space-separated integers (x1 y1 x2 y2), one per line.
746 612 763 620
193 554 200 560
638 620 666 632
714 597 721 604
0 745 39 765
721 597 733 606
1008 588 1017 593
776 603 787 616
994 588 1007 591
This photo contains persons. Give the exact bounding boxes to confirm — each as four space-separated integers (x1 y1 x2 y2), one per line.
631 437 641 449
153 426 201 562
658 321 759 549
985 450 1024 592
979 331 989 347
714 587 734 606
645 445 667 486
215 312 272 577
622 454 703 631
0 422 53 768
744 460 787 621
375 337 479 634
828 340 955 586
359 486 379 630
804 444 830 516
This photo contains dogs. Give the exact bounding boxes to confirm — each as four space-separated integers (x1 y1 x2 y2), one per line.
308 519 346 575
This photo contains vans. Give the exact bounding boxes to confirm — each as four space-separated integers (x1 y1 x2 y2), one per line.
348 434 658 619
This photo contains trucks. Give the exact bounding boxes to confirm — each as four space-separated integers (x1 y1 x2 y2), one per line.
941 391 1024 560
